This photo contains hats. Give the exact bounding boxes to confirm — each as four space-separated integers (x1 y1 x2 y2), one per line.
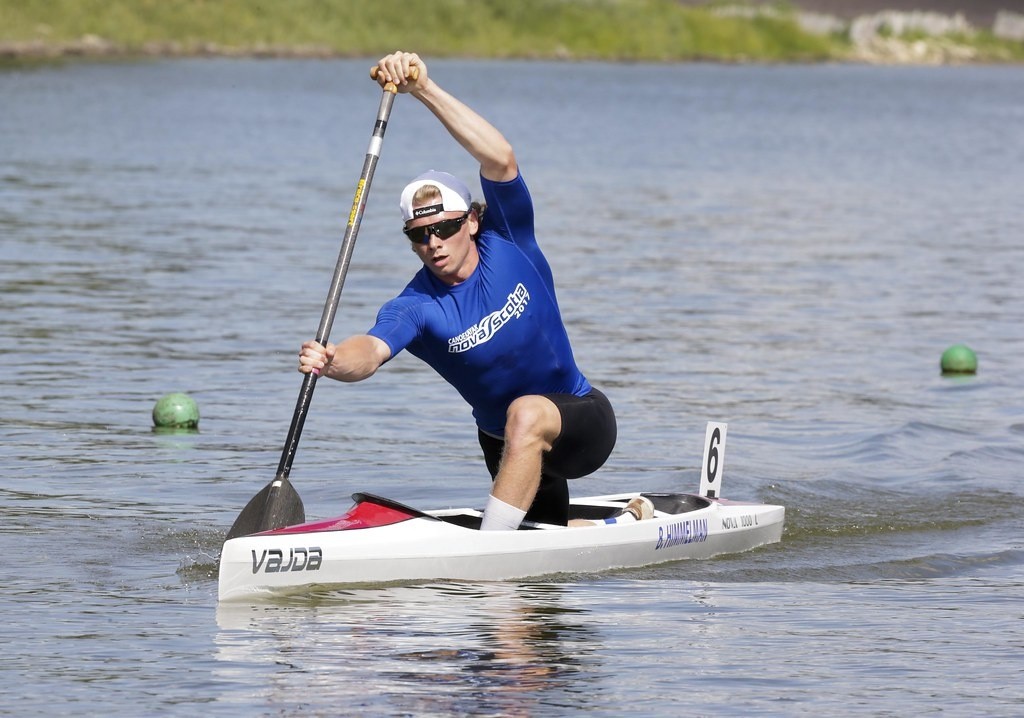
399 170 471 222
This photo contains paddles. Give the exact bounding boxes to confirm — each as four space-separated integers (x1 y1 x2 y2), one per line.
227 63 422 538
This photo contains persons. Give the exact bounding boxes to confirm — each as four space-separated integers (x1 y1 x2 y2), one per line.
298 49 618 532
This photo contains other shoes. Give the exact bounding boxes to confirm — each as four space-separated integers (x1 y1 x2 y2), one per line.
622 497 654 520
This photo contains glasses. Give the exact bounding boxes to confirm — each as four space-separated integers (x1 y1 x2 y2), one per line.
402 207 472 244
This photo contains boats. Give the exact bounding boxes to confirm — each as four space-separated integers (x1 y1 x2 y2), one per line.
218 491 786 591
